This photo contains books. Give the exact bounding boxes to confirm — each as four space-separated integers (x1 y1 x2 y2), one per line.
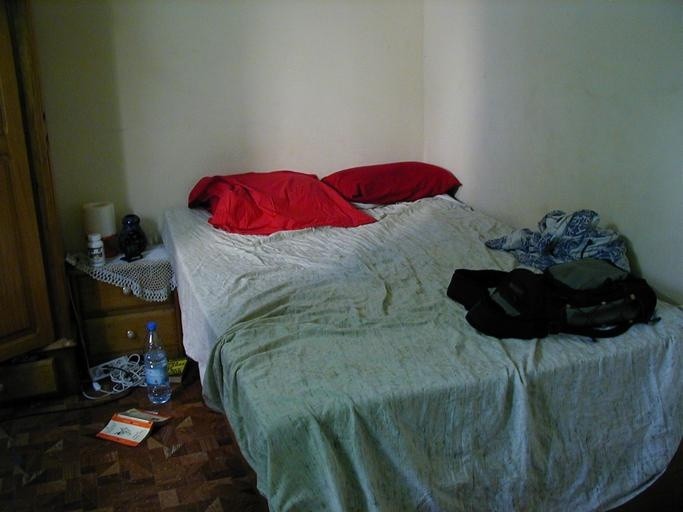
166 358 188 384
94 407 175 446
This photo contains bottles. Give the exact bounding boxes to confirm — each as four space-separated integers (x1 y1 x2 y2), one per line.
88 234 105 265
143 321 171 404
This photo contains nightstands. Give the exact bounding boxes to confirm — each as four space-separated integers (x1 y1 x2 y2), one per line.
68 244 185 378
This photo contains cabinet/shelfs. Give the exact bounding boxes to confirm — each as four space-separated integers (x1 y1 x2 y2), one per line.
1 0 86 406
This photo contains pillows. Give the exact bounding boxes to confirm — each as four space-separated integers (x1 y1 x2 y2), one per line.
321 161 461 205
188 171 376 236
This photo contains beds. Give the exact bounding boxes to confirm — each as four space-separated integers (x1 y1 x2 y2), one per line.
163 194 683 511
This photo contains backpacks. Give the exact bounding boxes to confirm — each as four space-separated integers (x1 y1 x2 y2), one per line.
446 256 662 342
